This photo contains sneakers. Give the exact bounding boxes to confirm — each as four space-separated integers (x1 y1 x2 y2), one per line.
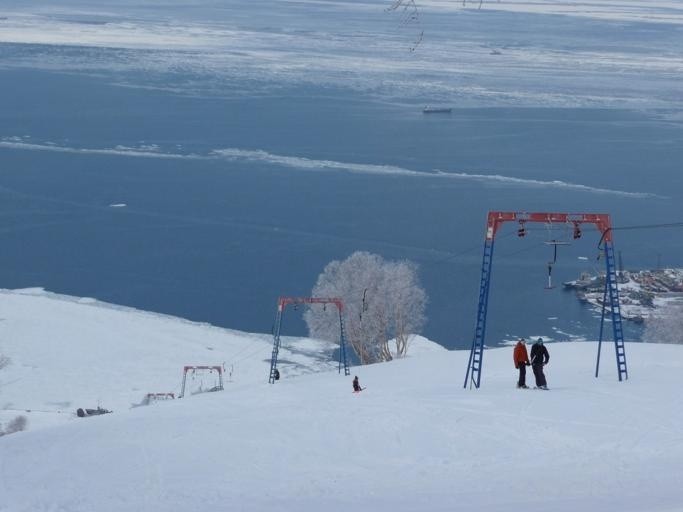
517 382 546 389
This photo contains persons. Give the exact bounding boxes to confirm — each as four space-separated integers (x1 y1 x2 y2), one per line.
530 337 549 389
352 375 360 391
274 369 280 380
513 338 530 388
